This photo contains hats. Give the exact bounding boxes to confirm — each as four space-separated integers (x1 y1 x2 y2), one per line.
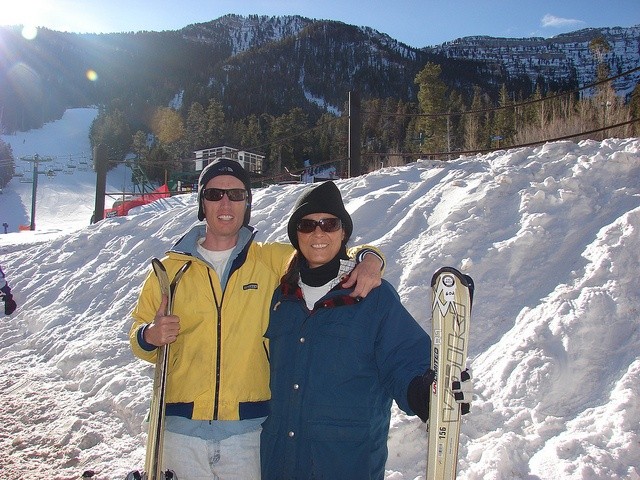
198 157 251 225
288 182 353 250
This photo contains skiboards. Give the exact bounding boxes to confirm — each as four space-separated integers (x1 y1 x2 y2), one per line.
143 257 194 480
427 266 471 480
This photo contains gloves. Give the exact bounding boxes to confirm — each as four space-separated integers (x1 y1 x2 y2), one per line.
410 370 475 423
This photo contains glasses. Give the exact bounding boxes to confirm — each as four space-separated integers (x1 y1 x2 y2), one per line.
201 189 247 202
297 218 342 233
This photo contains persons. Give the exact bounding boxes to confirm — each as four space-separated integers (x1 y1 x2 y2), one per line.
0 265 15 315
130 157 384 480
261 181 473 480
